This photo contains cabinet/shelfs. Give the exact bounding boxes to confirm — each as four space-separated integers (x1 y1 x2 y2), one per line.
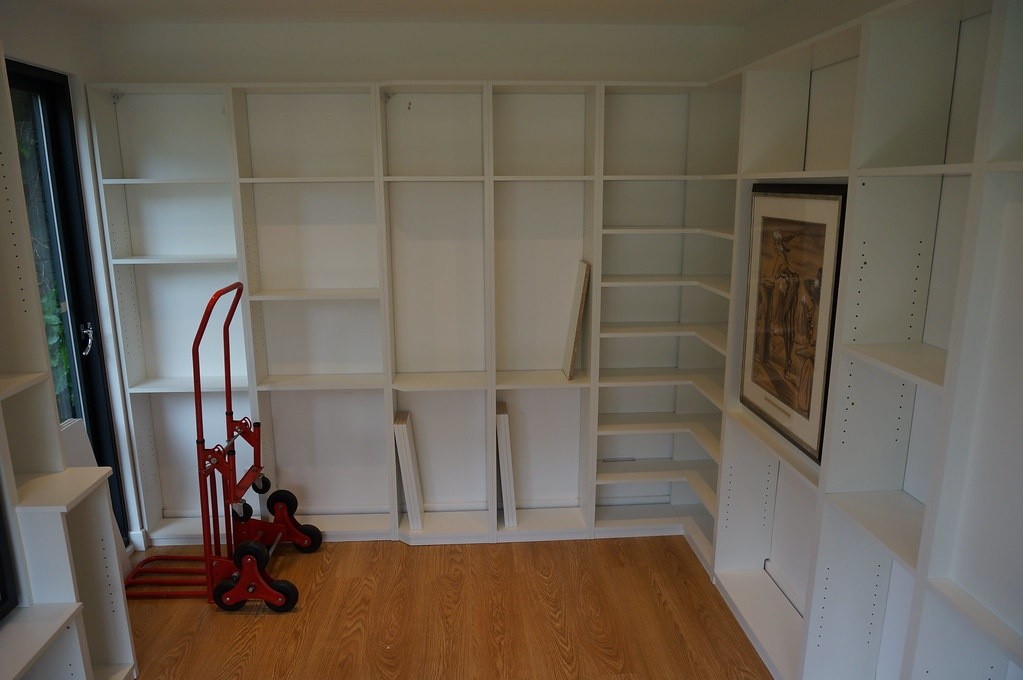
0 45 138 680
94 0 1023 680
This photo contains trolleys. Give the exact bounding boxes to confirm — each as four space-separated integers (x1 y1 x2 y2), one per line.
123 280 323 613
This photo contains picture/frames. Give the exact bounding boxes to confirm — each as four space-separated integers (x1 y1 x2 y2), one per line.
739 183 849 467
